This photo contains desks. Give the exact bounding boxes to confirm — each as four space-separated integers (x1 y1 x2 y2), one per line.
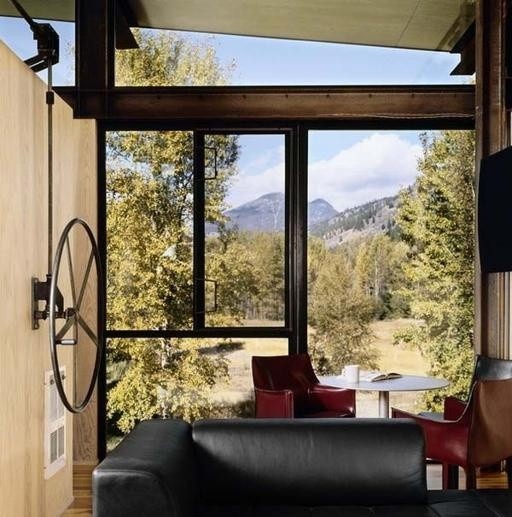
317 371 451 420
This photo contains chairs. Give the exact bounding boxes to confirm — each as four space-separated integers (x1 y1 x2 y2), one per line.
250 350 356 418
389 376 512 491
415 350 512 473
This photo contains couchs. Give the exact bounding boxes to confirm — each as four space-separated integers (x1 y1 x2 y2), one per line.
88 416 512 516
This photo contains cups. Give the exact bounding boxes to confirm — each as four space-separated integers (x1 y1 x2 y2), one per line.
341 364 361 383
